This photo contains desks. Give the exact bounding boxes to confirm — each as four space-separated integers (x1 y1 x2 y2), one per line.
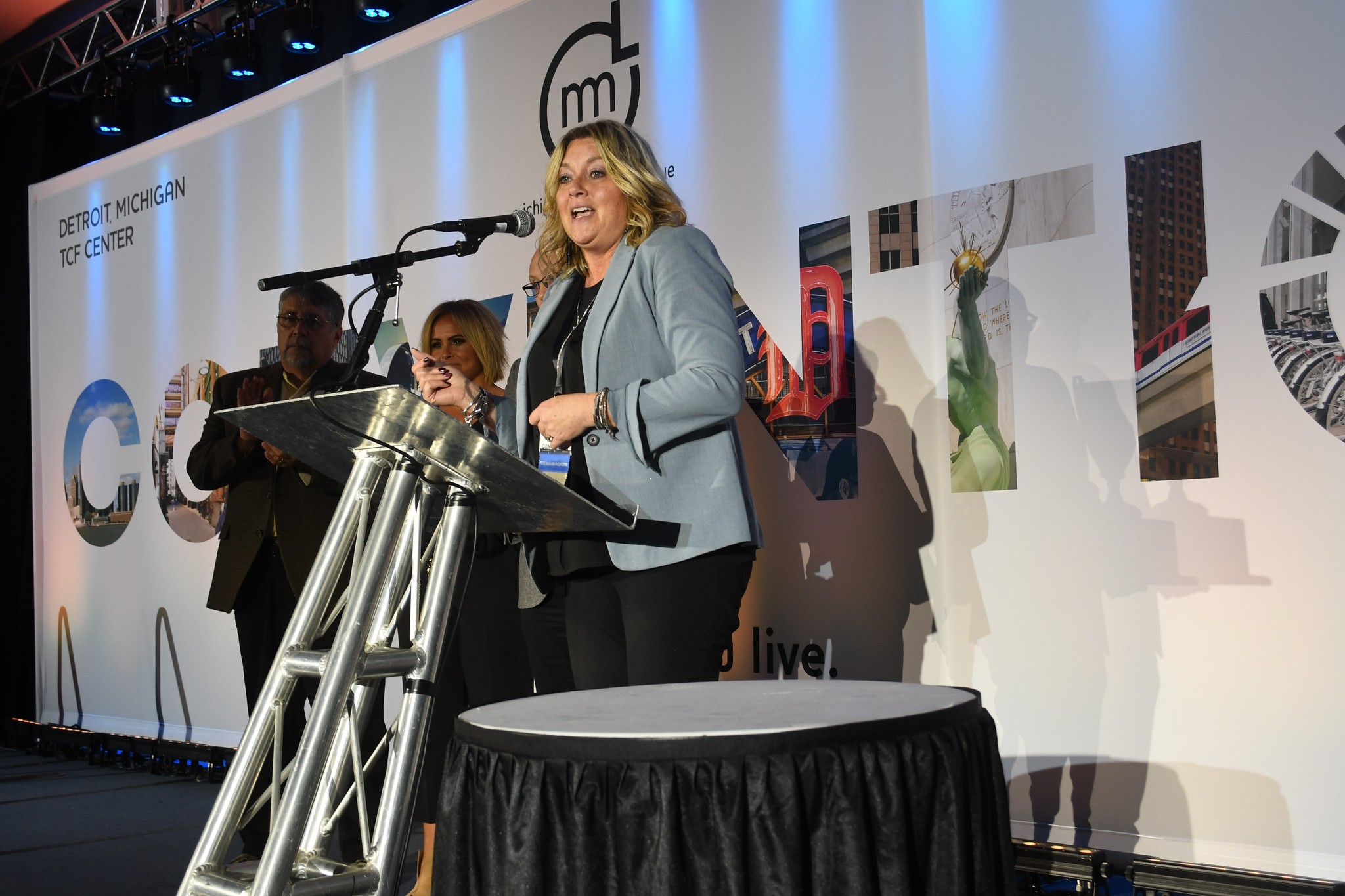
431 678 1015 896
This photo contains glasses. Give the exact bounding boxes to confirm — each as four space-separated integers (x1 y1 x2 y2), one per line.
522 273 560 297
276 314 339 330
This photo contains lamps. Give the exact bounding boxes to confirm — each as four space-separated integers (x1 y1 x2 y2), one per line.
222 10 260 82
281 0 324 55
81 59 131 138
356 0 399 24
158 17 198 109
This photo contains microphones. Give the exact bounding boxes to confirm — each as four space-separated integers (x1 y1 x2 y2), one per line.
431 207 538 238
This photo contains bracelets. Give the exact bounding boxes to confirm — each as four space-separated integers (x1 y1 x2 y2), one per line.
462 386 488 428
593 387 616 439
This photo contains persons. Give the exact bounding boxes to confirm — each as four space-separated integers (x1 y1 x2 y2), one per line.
408 119 773 691
168 486 179 511
186 280 394 865
501 241 578 696
406 298 535 895
946 263 1010 494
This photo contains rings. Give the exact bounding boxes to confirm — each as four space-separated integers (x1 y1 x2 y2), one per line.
543 433 553 442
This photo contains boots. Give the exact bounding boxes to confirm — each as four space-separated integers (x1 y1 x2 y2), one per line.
404 850 434 896
